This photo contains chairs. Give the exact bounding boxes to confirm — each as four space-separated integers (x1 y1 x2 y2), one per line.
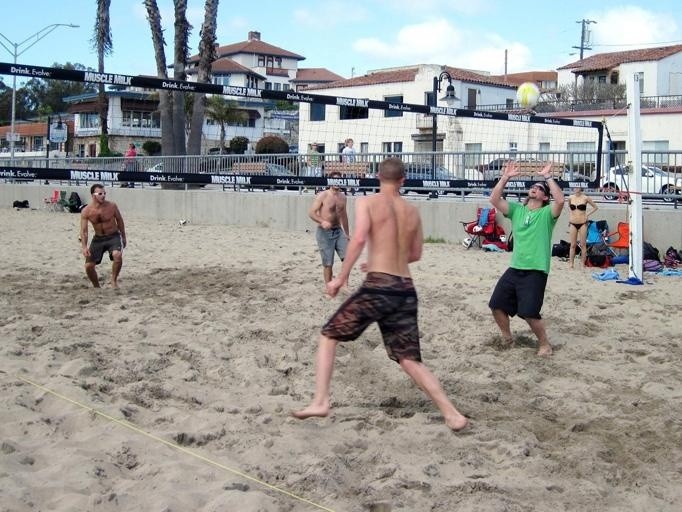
459 207 496 249
603 221 629 256
45 190 68 213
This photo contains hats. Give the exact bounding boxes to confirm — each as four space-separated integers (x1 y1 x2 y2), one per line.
472 225 482 232
462 238 472 249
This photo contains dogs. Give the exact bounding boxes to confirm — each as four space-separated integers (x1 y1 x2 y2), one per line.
12 199 30 209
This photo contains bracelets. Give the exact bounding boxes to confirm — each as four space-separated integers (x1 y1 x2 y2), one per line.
503 174 511 179
544 175 553 180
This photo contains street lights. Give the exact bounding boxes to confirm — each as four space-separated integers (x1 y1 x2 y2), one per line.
0 21 80 173
428 69 459 197
43 113 67 187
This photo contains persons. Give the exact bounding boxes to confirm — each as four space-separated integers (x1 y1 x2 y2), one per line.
288 157 467 430
81 184 126 291
567 188 598 268
309 172 353 293
125 143 136 187
342 139 356 178
490 159 564 357
302 143 321 192
340 139 348 163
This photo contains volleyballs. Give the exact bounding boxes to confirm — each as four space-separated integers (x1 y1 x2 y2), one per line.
472 225 483 233
179 219 187 226
516 82 540 108
463 238 474 248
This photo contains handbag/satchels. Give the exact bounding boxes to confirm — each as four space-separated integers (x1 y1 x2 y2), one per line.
585 255 609 268
551 240 581 257
643 259 662 271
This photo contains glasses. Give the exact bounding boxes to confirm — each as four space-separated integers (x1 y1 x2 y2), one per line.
92 192 105 195
530 185 547 195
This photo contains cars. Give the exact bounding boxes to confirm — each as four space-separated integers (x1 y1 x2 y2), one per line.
145 152 303 185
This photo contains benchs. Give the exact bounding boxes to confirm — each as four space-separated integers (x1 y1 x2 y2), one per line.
323 160 370 195
670 186 682 209
223 162 269 192
497 161 565 201
